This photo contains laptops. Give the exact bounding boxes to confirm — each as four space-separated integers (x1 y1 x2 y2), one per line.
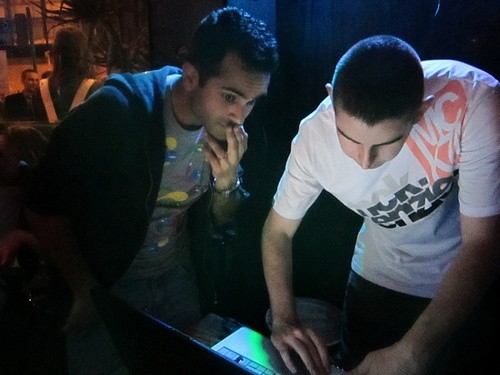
90 285 345 375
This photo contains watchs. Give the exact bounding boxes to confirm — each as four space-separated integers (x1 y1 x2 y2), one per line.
212 178 240 200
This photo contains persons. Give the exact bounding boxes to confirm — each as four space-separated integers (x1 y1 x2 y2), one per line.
0 22 104 375
259 35 500 375
0 6 277 375
